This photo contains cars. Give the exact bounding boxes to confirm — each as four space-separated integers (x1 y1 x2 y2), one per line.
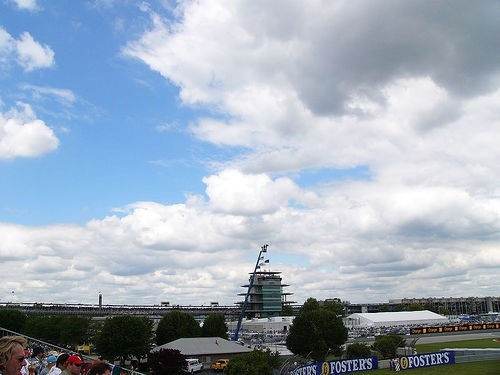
131 360 138 369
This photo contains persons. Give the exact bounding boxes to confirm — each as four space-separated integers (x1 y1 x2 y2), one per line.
19 345 127 374
1 334 28 374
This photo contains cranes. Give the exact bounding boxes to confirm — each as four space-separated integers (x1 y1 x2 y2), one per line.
228 244 268 345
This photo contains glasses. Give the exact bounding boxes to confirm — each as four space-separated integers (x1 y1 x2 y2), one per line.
17 356 28 362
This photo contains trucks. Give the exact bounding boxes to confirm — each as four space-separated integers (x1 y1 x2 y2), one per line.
396 347 418 357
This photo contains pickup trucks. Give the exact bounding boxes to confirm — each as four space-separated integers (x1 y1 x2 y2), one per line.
211 359 230 373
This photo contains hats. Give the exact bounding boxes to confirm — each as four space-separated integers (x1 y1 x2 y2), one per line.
47 355 57 363
66 356 85 365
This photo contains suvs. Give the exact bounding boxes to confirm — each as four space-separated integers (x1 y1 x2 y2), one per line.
185 359 204 375
